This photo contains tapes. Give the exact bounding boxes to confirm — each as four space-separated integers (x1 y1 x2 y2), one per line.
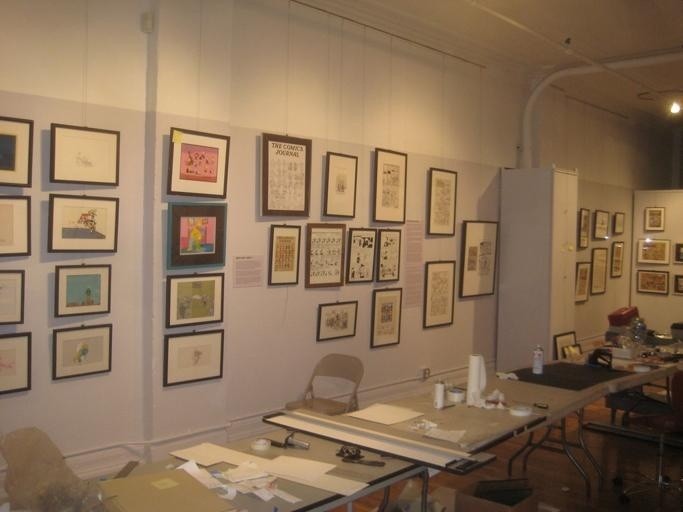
446 389 465 402
509 405 532 416
250 438 271 450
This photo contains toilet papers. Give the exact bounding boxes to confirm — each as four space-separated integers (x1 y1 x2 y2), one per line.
466 353 487 408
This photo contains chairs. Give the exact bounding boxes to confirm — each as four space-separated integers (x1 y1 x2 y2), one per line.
611 365 683 504
285 353 363 415
0 427 84 511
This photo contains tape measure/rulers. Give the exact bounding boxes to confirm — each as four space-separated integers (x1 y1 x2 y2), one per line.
342 458 385 467
112 461 139 479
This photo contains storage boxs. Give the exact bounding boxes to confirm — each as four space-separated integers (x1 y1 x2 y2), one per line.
413 472 540 512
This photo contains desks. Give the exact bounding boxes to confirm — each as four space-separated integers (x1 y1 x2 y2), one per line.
342 377 609 512
504 347 683 493
88 429 428 512
658 355 683 372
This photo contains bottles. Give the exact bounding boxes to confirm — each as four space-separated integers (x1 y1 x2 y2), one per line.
432 377 446 410
630 317 647 343
531 344 545 375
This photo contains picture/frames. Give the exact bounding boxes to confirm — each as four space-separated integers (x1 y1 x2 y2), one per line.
167 202 226 267
316 300 360 341
377 228 401 282
260 130 312 217
304 221 346 287
323 150 358 220
164 330 225 386
423 261 455 328
373 147 408 224
370 287 401 348
426 167 457 237
562 344 583 358
459 220 497 298
575 207 683 303
165 272 226 327
0 115 120 394
348 227 377 284
165 127 231 199
553 331 577 360
268 224 300 287
